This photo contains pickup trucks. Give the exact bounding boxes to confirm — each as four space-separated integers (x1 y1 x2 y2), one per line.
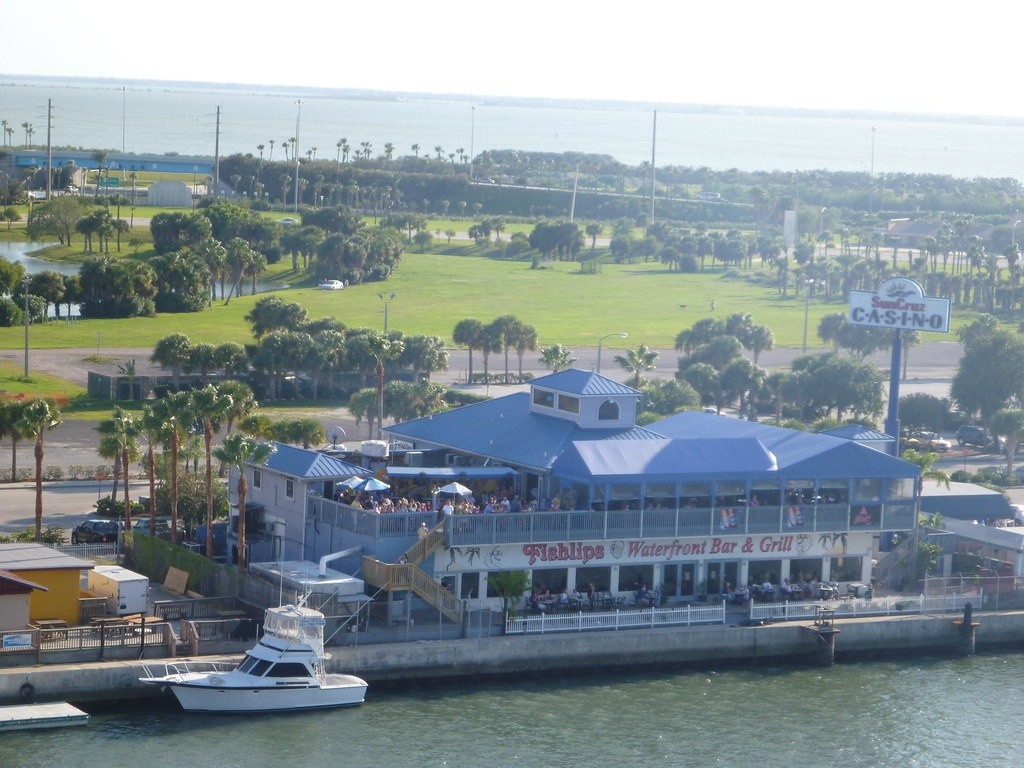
131 518 187 545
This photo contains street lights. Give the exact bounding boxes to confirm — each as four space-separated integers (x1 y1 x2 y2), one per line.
293 99 306 213
121 85 127 155
598 332 628 375
22 275 32 377
378 292 396 332
819 207 827 253
470 104 476 178
1013 220 1022 245
803 279 814 353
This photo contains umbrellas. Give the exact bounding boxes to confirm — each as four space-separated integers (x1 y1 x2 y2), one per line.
439 482 471 502
336 476 363 490
354 476 391 491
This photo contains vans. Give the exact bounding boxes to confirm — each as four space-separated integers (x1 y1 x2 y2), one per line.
696 192 720 201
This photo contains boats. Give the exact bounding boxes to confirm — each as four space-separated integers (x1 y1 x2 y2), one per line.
138 559 369 713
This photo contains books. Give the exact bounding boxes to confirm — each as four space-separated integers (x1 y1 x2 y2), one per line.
442 492 578 531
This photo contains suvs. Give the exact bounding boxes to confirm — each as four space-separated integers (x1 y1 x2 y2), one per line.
71 520 118 543
64 185 79 193
957 424 1000 447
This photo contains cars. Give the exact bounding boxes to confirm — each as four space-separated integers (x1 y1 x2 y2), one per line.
276 217 299 224
475 177 495 183
900 428 952 453
320 280 343 290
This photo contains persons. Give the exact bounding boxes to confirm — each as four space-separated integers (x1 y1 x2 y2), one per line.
417 522 428 558
751 494 760 507
336 486 431 533
531 581 595 612
397 554 409 583
725 576 838 605
635 585 662 608
592 495 730 527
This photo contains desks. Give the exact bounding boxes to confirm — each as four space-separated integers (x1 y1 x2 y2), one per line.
821 585 833 598
541 602 554 612
793 589 802 601
604 597 615 604
645 597 657 608
851 582 864 598
35 620 69 641
733 592 743 604
129 617 165 638
216 610 248 620
764 588 774 603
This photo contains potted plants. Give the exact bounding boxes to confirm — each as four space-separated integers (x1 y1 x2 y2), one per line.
696 581 708 602
659 583 672 603
915 542 945 594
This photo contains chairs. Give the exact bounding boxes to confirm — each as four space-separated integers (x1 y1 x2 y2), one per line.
711 591 721 605
847 584 855 596
524 592 642 612
